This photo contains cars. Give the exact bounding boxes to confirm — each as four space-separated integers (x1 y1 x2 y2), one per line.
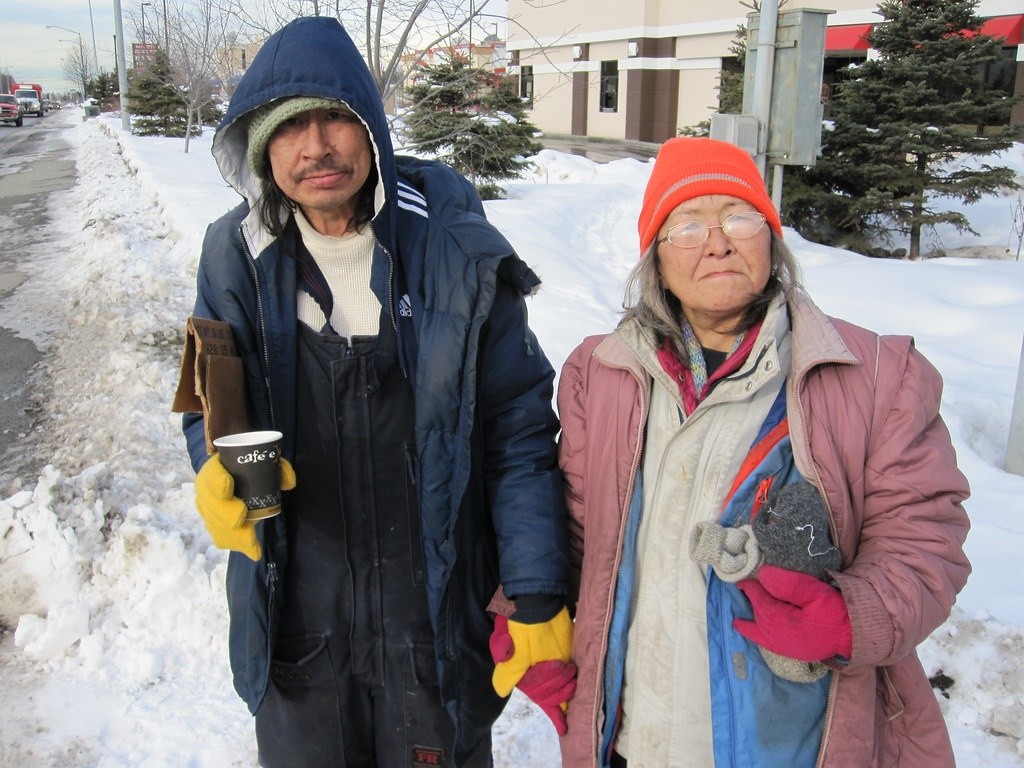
43 100 63 112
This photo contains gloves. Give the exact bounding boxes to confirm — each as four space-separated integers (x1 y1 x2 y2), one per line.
492 618 575 736
490 605 575 712
733 564 852 663
194 451 296 561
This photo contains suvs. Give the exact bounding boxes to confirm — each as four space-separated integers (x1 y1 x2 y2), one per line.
0 94 24 127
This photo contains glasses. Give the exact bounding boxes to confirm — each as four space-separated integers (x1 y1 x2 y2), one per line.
656 212 768 249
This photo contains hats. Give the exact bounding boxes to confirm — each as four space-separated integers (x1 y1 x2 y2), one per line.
247 93 356 179
638 138 783 266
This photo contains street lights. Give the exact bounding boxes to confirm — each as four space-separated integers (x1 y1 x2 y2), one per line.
491 22 498 39
141 2 152 44
46 26 86 106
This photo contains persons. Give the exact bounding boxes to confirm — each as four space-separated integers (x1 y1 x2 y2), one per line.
486 137 972 768
183 17 575 768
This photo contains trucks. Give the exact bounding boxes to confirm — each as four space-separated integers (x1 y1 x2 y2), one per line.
11 83 44 117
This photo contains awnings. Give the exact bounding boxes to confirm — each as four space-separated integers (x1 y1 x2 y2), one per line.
825 24 875 51
916 13 1024 48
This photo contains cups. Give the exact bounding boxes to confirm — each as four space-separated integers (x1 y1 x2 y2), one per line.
213 431 284 521
